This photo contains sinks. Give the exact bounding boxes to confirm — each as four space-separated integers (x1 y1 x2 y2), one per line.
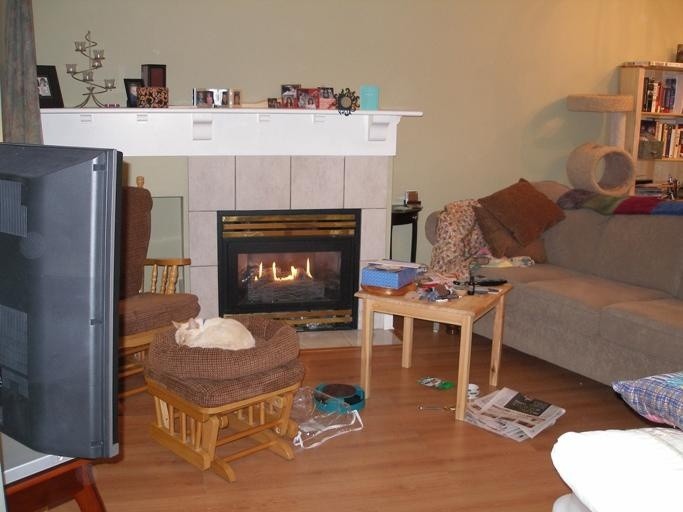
425 180 682 395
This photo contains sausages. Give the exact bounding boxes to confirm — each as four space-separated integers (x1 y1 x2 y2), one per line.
171 317 256 350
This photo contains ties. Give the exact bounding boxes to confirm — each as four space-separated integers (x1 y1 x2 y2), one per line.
0 142 123 486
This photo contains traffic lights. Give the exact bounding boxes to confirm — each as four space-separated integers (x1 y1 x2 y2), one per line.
471 204 549 264
482 177 568 242
550 371 682 511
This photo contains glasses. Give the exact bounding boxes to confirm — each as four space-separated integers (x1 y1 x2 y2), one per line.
64 28 117 109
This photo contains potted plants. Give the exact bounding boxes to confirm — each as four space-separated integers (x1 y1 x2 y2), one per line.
191 87 240 108
35 62 64 108
122 78 146 107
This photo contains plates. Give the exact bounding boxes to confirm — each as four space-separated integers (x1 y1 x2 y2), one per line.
615 53 682 164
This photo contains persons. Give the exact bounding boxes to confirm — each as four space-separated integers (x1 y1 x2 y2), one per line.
196 91 204 105
129 81 140 106
327 88 333 98
285 97 292 107
319 89 323 98
205 92 212 105
233 92 240 105
283 85 295 98
322 89 329 98
37 77 50 96
305 97 314 107
297 92 304 107
221 93 228 105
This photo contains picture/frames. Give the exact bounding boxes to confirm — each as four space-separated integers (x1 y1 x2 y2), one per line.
351 278 515 423
3 457 109 512
389 203 424 266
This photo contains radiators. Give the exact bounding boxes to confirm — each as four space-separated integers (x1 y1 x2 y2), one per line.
454 279 508 286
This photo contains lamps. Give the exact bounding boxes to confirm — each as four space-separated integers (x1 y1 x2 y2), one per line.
119 175 201 401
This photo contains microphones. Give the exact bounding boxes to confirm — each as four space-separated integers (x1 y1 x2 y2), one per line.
141 314 309 484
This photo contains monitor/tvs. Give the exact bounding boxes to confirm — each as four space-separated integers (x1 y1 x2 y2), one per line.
642 76 683 160
366 256 428 275
